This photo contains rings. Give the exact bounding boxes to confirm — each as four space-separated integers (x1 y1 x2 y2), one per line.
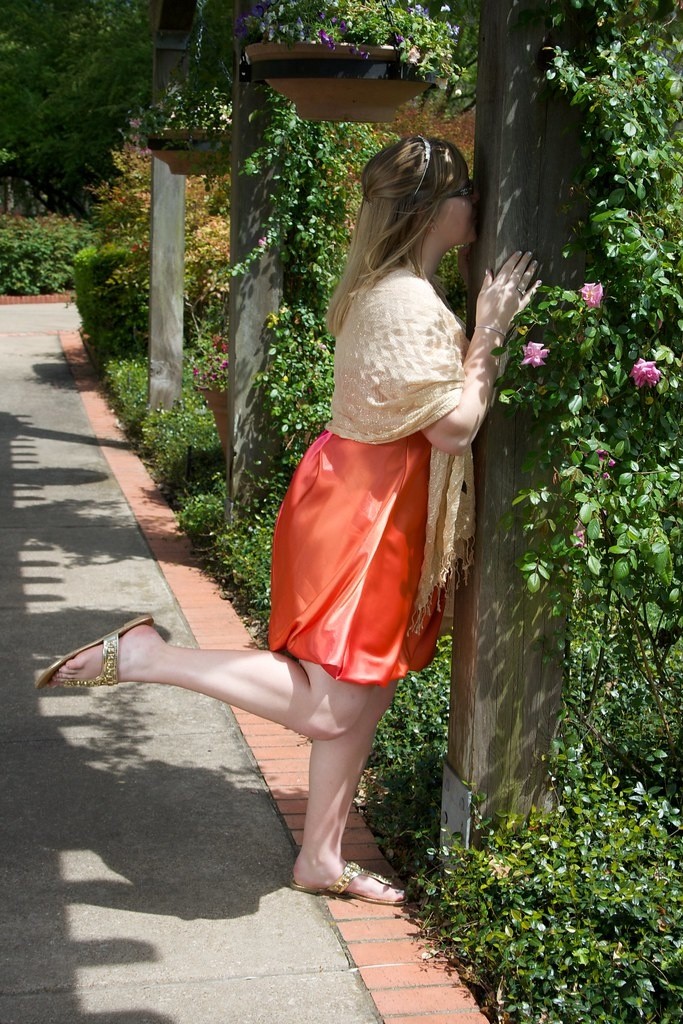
516 287 526 296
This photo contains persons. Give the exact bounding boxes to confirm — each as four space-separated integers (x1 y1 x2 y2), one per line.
35 136 542 906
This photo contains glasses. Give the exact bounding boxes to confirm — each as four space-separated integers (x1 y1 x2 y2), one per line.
444 180 474 199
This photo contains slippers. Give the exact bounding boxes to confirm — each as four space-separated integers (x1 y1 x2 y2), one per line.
290 860 408 906
35 615 154 689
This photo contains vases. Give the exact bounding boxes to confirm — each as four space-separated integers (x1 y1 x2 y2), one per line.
245 44 435 123
201 389 227 457
146 126 225 176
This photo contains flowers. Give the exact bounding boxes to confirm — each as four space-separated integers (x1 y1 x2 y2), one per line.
122 83 232 218
233 0 470 97
192 334 228 394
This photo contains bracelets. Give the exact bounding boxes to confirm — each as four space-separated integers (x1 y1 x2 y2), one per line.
474 326 506 338
